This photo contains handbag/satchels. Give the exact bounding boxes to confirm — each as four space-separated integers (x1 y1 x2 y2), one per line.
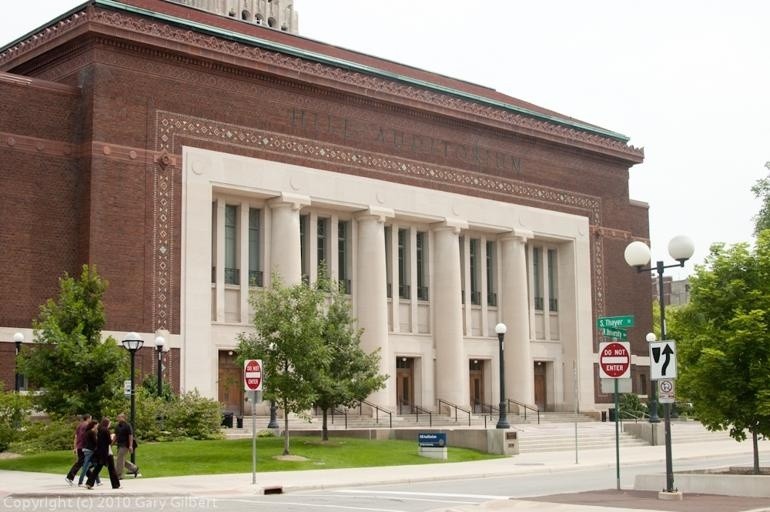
128 439 137 449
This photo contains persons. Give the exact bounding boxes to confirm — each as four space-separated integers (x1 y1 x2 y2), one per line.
66 414 92 486
111 414 138 480
78 421 103 487
85 418 120 490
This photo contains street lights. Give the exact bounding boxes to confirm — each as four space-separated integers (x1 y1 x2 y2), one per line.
122 329 145 472
12 331 24 428
493 322 510 429
154 338 165 402
645 332 661 424
624 234 696 493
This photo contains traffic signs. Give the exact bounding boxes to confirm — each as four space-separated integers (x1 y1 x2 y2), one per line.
595 316 636 328
603 326 628 341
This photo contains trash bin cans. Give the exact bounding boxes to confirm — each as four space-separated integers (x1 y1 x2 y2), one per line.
609 409 615 421
602 412 606 422
236 416 243 428
221 411 233 428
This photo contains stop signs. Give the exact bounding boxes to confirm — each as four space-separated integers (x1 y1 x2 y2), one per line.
245 360 263 392
597 341 632 379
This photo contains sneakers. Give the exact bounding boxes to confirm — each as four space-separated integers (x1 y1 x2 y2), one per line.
65 477 73 485
79 484 84 487
97 483 103 485
134 467 138 478
85 485 94 490
